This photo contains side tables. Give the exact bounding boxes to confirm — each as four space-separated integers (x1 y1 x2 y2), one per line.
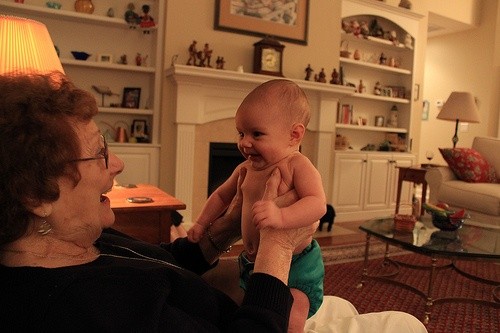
394 166 428 217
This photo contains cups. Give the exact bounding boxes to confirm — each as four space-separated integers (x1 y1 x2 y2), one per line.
116 127 128 143
375 116 384 126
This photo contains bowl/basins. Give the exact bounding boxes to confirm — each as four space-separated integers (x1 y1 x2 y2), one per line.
71 51 91 60
431 212 471 231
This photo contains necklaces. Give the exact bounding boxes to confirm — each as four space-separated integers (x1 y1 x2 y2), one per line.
1 243 183 272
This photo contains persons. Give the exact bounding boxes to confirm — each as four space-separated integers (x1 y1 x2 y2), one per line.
185 40 225 70
186 79 327 333
304 64 338 84
0 72 429 333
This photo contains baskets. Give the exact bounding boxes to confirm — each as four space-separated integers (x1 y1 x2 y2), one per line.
395 203 417 234
430 212 471 231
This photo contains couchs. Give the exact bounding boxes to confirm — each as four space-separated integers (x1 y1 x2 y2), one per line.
423 135 500 254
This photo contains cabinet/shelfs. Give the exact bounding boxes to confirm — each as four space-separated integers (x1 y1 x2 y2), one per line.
0 0 160 188
332 152 417 225
338 0 421 149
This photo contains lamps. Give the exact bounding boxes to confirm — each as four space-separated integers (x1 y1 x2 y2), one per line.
0 14 68 86
434 90 481 147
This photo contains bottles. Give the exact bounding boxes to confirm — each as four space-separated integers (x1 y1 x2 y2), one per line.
389 106 399 128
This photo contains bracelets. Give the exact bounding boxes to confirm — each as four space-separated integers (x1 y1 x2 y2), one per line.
205 220 231 253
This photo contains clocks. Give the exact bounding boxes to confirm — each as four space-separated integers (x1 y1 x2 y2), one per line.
252 33 286 79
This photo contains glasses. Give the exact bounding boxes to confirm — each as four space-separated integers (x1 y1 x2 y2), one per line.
62 135 110 169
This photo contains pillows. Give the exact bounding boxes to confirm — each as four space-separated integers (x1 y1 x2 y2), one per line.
437 146 498 183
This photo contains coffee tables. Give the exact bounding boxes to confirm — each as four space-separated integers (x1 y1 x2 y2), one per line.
359 212 500 324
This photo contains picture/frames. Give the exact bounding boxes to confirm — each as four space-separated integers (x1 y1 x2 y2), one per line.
213 0 311 47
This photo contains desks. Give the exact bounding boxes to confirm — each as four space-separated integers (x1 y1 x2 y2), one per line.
102 184 187 243
